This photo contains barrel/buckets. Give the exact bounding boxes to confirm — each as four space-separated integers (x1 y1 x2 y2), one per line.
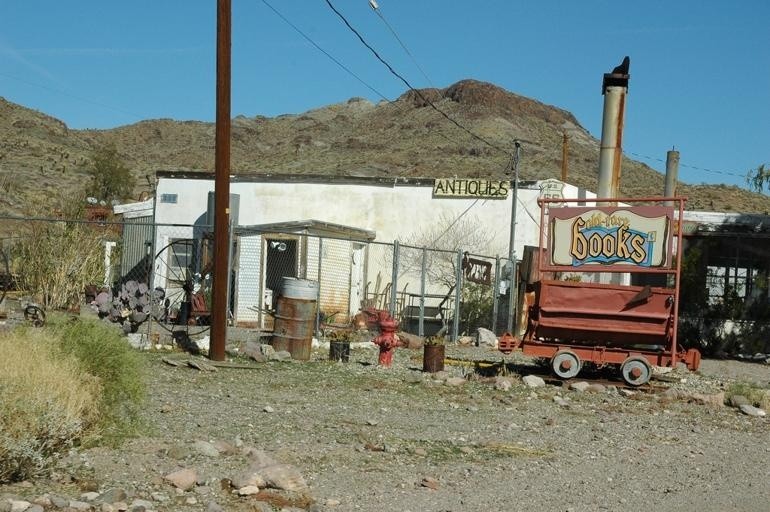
281 277 320 300
271 298 317 361
423 345 444 372
329 341 350 363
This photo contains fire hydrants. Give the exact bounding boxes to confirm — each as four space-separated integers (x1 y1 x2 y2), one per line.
370 318 404 368
